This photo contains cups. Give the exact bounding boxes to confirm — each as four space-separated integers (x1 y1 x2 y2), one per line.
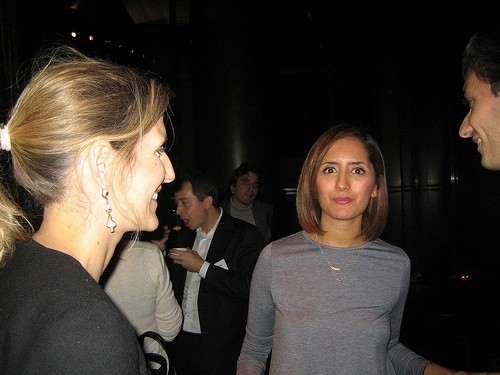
174 248 187 263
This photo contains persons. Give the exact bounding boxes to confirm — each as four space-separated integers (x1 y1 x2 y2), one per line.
459 8 500 171
151 172 266 375
0 45 177 375
99 240 184 375
215 164 272 244
236 124 471 375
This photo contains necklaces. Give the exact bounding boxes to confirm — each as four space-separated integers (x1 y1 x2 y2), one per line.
316 233 367 286
